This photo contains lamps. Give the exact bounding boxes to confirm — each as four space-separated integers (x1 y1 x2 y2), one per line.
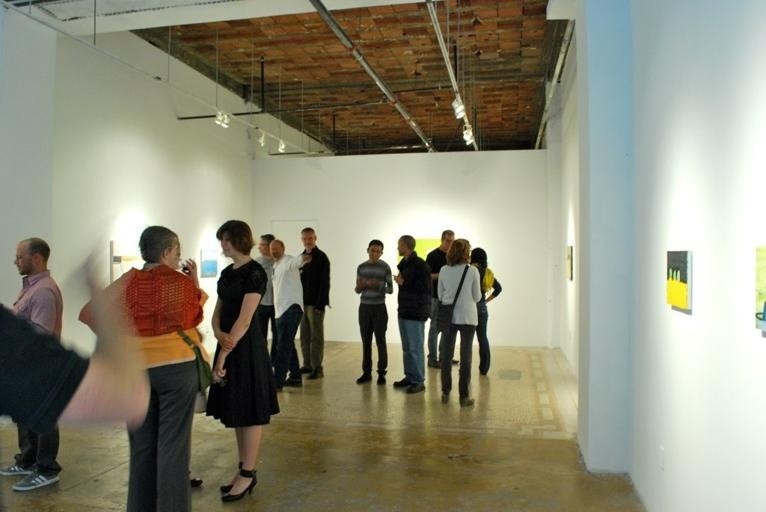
452 100 465 118
463 124 474 144
215 111 231 128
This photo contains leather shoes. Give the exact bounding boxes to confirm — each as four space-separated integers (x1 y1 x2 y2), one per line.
283 378 302 387
428 359 440 367
406 384 425 393
274 382 283 392
357 374 372 383
394 379 415 387
452 360 459 363
377 375 386 385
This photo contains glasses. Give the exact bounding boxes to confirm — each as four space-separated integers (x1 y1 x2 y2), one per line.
259 242 268 246
444 238 454 242
370 247 383 254
168 243 178 250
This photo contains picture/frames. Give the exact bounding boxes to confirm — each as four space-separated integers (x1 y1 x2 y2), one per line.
564 245 572 280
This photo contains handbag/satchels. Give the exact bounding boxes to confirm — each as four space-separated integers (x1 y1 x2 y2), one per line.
430 263 471 337
194 391 207 413
177 329 213 392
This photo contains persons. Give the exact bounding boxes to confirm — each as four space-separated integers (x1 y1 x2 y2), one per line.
437 239 478 408
2 238 64 492
0 277 152 429
99 224 201 512
206 220 280 501
394 236 430 395
423 230 461 370
467 248 502 374
268 242 314 393
297 229 330 379
356 240 393 386
254 235 284 367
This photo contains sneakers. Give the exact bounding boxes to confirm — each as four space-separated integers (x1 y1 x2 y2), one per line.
12 469 60 491
300 366 312 373
307 366 323 379
479 373 489 385
0 462 38 476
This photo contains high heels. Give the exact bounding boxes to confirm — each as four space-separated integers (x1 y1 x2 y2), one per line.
188 471 203 488
220 461 243 493
221 469 257 502
460 396 473 408
441 393 449 404
